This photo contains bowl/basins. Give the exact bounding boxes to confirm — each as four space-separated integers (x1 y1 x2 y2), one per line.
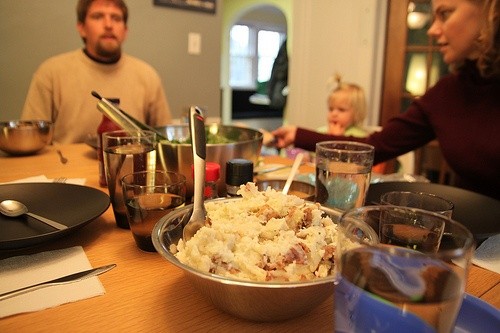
153 195 380 321
255 179 316 200
0 119 54 155
154 124 264 175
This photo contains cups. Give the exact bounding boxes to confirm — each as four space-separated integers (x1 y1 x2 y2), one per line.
380 192 455 221
121 170 186 253
312 141 376 212
332 205 477 332
102 130 157 229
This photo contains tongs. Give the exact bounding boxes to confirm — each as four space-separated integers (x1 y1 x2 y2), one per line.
95 97 153 137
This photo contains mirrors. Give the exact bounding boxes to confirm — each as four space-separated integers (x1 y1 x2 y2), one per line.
216 0 291 135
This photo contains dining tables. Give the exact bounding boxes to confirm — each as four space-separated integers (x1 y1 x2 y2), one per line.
0 141 500 333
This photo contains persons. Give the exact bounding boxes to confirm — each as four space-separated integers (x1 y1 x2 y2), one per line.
20 0 172 145
264 0 500 201
315 75 369 140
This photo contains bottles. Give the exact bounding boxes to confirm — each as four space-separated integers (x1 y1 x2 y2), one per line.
190 162 222 202
226 158 254 198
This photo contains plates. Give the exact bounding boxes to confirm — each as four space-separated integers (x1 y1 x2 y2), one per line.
0 184 112 247
451 293 500 333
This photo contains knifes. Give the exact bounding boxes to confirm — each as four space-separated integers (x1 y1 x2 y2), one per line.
57 150 68 164
0 263 116 301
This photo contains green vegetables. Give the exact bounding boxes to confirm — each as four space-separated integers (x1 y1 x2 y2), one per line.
171 129 238 144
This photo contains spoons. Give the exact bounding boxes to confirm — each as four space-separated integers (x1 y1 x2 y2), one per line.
0 199 69 232
183 106 212 241
